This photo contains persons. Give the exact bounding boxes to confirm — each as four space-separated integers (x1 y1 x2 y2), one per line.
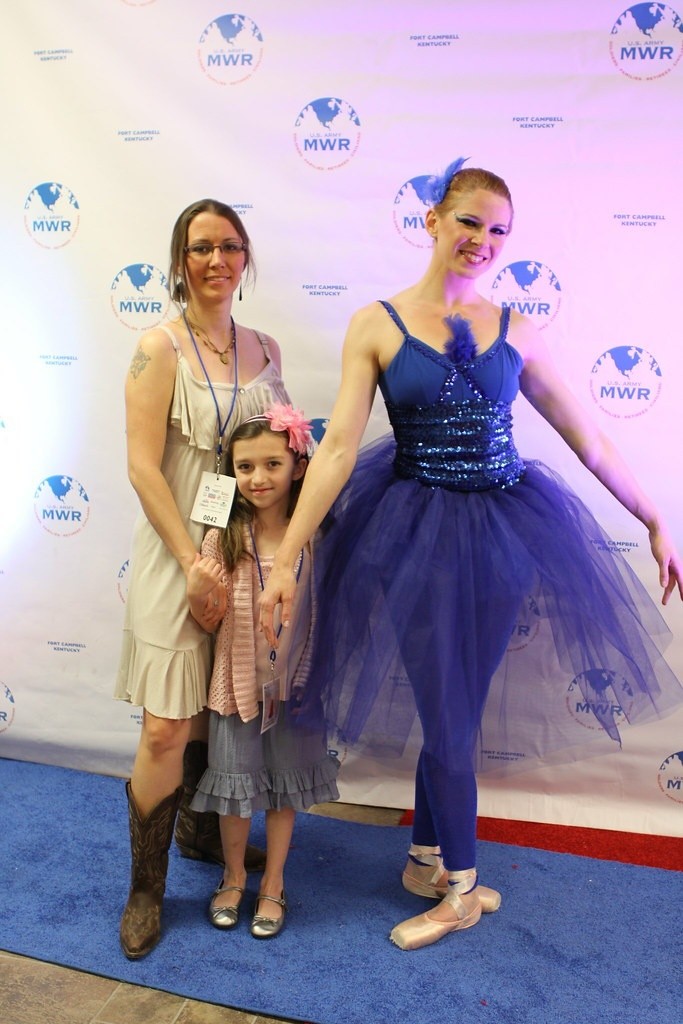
122 199 291 959
258 157 683 949
187 407 336 938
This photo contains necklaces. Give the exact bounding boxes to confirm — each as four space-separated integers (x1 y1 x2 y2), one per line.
187 317 236 364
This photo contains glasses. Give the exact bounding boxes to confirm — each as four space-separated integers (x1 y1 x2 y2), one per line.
183 241 247 260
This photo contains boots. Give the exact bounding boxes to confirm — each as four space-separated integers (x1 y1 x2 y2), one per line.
175 741 268 870
120 778 183 957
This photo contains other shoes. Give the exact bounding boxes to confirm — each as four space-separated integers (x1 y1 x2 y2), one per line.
250 887 285 937
402 843 502 913
209 879 247 927
389 867 483 951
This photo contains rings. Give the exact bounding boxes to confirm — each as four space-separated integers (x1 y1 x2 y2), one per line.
215 601 219 606
